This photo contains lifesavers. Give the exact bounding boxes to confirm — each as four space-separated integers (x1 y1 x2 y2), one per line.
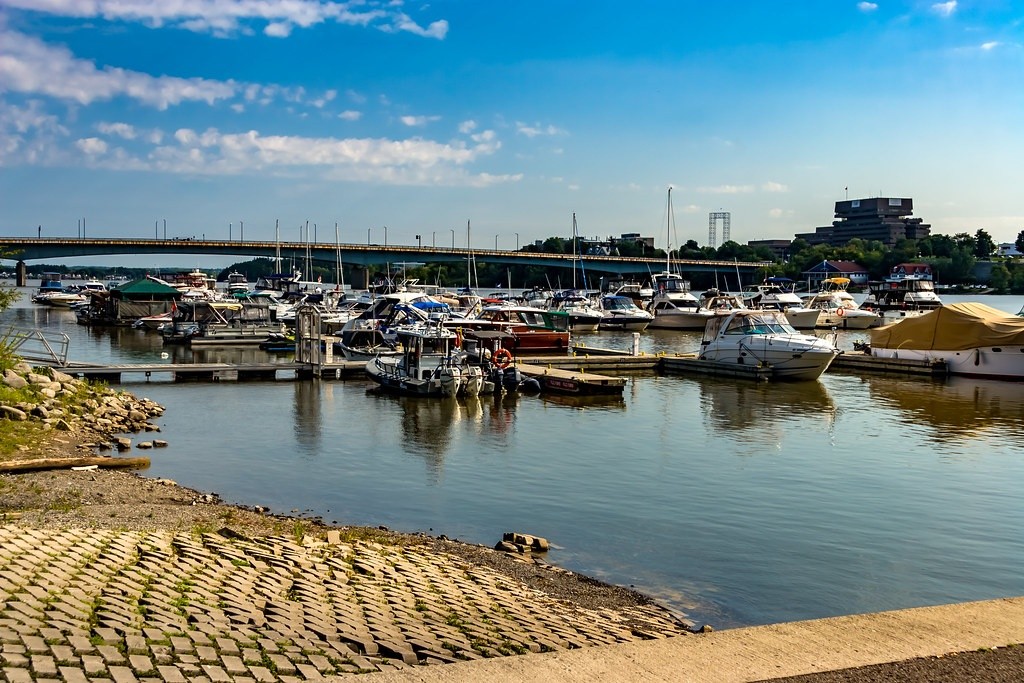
493 349 512 369
867 307 872 312
837 308 843 316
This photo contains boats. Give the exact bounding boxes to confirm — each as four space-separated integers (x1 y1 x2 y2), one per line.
365 266 495 398
696 309 845 381
485 365 541 394
30 186 944 350
852 301 1024 379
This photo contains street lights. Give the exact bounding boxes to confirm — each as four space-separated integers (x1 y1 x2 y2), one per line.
314 224 316 245
450 230 454 251
78 220 81 239
83 216 86 240
300 226 303 244
495 234 499 252
433 232 436 249
384 227 387 247
240 221 244 243
156 221 159 240
368 228 371 247
515 233 518 254
230 223 232 241
163 219 166 241
